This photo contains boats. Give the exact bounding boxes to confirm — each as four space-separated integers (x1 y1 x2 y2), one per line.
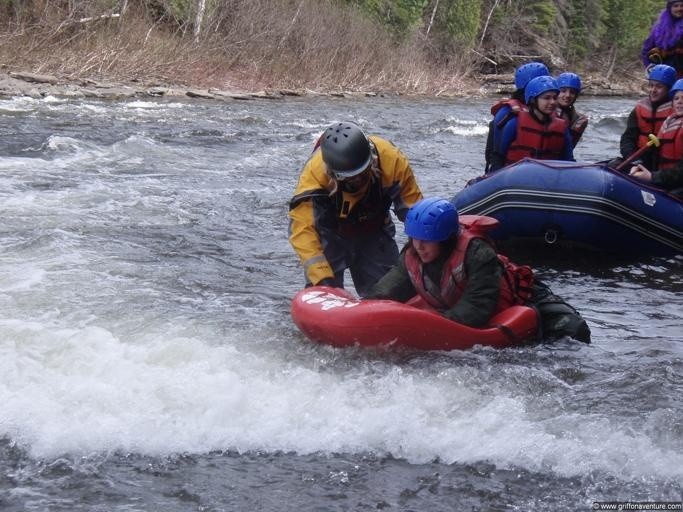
292 283 539 357
442 157 683 266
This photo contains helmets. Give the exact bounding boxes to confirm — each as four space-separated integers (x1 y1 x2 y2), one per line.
320 120 374 180
515 61 561 106
646 63 683 98
403 195 459 242
556 72 582 92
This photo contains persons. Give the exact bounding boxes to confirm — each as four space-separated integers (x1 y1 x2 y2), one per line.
640 0 683 80
484 63 588 175
366 197 591 346
288 122 425 299
609 64 677 174
629 78 683 203
